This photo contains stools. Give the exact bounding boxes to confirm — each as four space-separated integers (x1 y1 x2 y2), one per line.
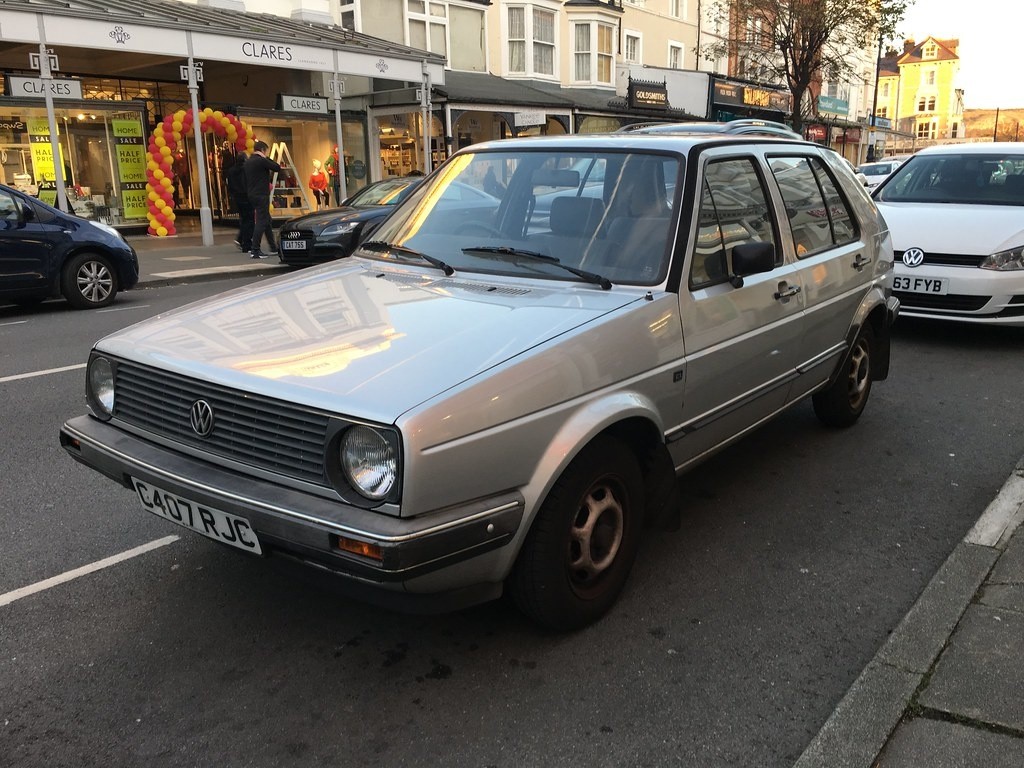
313 195 323 211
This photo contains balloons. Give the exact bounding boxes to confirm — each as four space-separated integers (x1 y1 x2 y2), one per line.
146 108 274 236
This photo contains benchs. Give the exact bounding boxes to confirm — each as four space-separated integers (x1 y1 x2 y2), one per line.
982 166 1005 192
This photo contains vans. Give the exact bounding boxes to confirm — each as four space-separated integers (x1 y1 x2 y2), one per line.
0 183 140 310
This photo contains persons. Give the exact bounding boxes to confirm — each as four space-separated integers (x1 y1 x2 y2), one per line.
208 141 227 215
325 144 350 206
222 141 234 213
175 150 192 207
246 141 281 259
228 152 255 253
309 159 329 211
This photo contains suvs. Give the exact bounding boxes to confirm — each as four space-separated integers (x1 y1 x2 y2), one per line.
492 116 807 245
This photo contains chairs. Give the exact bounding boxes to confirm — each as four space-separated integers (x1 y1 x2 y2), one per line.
594 186 674 275
1001 174 1024 202
526 196 609 268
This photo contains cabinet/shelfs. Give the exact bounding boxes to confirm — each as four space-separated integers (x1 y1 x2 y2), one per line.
268 142 314 219
380 134 448 178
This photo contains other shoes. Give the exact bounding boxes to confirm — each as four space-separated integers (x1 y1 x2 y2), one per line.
270 249 280 255
250 249 269 259
234 237 242 247
242 249 250 255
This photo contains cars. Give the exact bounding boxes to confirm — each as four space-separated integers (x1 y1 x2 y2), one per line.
869 142 1024 340
855 160 903 196
276 175 507 267
56 131 901 635
